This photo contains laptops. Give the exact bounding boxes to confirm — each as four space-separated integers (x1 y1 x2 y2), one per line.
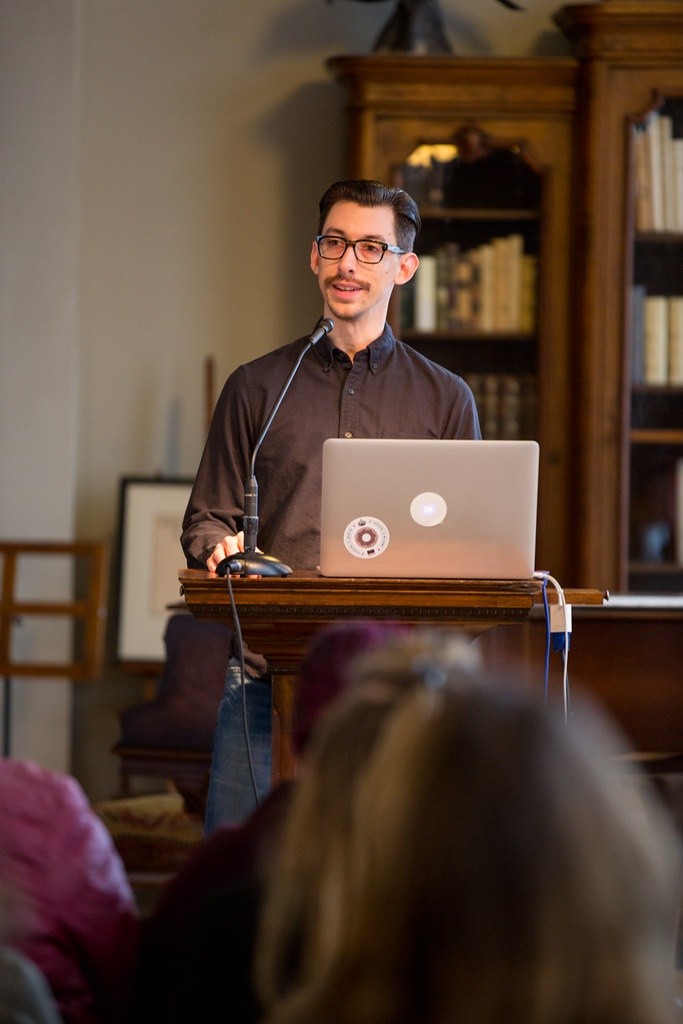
316 437 539 582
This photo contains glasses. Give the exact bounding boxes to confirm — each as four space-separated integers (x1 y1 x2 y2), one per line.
316 235 405 264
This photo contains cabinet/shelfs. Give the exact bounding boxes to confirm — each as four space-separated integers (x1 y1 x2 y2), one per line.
324 0 683 598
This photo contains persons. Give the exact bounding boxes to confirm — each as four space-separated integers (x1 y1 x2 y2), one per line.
181 179 482 832
0 621 683 1024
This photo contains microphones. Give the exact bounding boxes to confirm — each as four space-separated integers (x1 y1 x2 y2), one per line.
215 319 334 576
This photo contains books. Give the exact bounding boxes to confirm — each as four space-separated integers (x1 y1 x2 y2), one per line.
460 370 541 438
630 288 683 388
631 110 683 234
395 235 539 334
